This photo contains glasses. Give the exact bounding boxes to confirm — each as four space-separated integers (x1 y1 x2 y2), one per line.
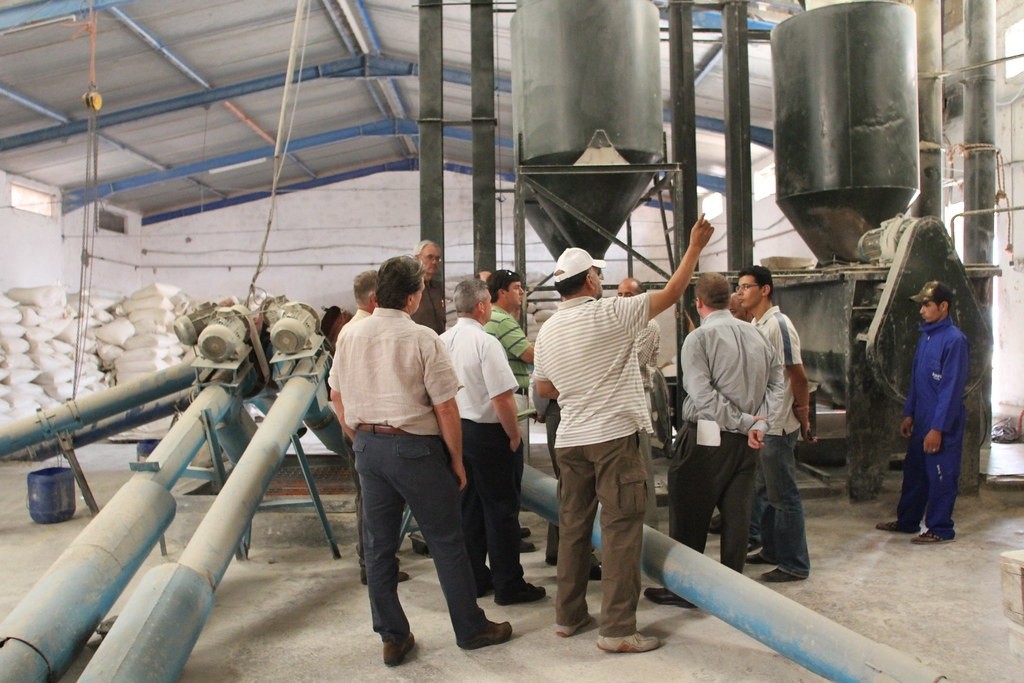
417 254 442 262
500 270 511 289
736 283 762 291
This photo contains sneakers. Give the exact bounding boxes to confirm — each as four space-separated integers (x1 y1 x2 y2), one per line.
457 620 512 649
383 631 414 666
598 631 660 651
556 610 592 637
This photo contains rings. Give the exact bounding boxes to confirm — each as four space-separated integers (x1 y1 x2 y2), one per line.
932 449 936 451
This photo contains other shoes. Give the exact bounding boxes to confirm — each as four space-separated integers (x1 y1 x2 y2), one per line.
588 565 602 581
545 557 557 564
519 527 531 538
519 540 535 554
495 582 545 605
480 571 493 596
361 557 409 584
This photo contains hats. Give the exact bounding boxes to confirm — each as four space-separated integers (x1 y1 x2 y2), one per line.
554 247 605 282
908 281 952 303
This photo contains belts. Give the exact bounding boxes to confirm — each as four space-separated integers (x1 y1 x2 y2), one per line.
358 424 409 435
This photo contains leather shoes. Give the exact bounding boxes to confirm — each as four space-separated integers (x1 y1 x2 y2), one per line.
746 553 768 565
644 587 697 609
761 569 797 582
747 537 761 551
709 513 720 533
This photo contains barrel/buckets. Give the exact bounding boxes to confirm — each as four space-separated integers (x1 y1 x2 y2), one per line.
26 467 77 524
135 440 161 461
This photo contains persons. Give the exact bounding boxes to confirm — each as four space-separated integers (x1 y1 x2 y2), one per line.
410 240 446 336
341 269 409 585
735 265 818 583
437 280 546 605
532 385 602 580
617 278 661 531
531 213 714 653
483 270 535 552
876 281 969 545
708 292 763 552
643 271 784 609
327 255 512 667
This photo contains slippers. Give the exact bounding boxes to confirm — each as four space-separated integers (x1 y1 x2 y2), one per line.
912 532 955 544
875 520 913 533
